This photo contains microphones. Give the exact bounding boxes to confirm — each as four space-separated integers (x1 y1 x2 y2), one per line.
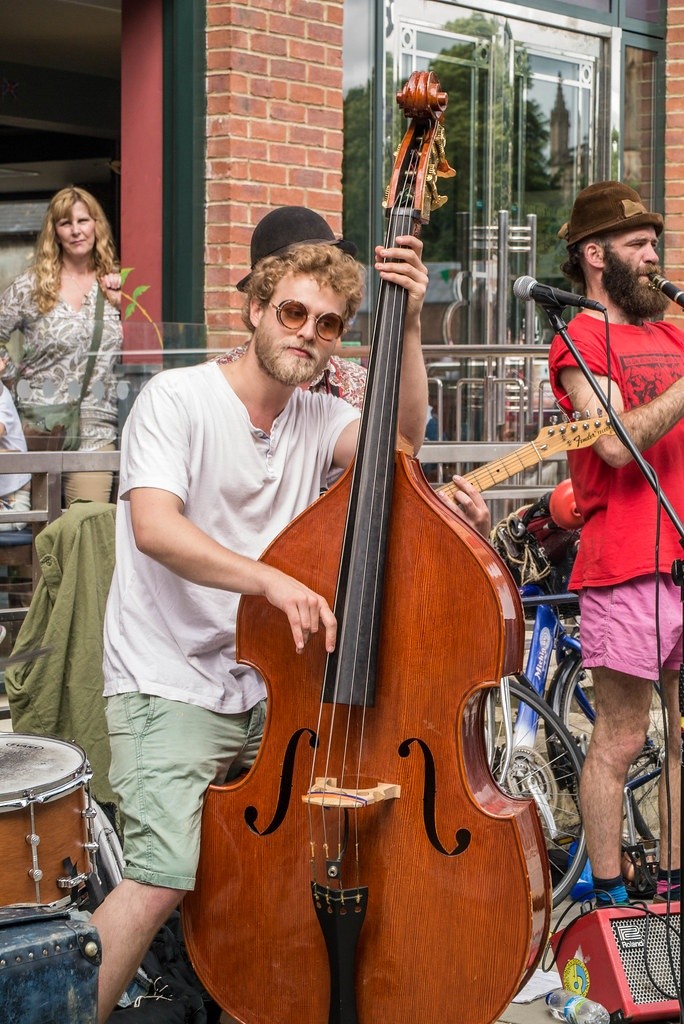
513 275 607 313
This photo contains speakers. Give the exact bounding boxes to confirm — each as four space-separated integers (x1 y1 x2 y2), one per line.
549 902 682 1024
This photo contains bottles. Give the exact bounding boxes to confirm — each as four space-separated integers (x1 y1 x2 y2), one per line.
545 992 610 1024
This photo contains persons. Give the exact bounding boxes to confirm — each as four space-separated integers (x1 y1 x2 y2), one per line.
91 204 492 1024
548 182 684 909
0 187 124 533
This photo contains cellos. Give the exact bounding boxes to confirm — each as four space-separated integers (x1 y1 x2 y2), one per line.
178 71 552 1024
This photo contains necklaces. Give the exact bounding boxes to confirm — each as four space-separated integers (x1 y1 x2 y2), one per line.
64 265 91 305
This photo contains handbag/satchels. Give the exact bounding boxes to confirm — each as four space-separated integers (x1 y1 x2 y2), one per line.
16 401 83 451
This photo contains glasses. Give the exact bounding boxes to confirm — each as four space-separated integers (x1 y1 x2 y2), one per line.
268 299 347 342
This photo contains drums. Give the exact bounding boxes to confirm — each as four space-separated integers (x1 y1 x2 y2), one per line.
0 729 99 928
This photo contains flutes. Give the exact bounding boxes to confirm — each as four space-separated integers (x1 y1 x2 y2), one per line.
650 267 684 308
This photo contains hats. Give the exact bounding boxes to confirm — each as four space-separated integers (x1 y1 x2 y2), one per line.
236 206 358 292
566 180 663 249
